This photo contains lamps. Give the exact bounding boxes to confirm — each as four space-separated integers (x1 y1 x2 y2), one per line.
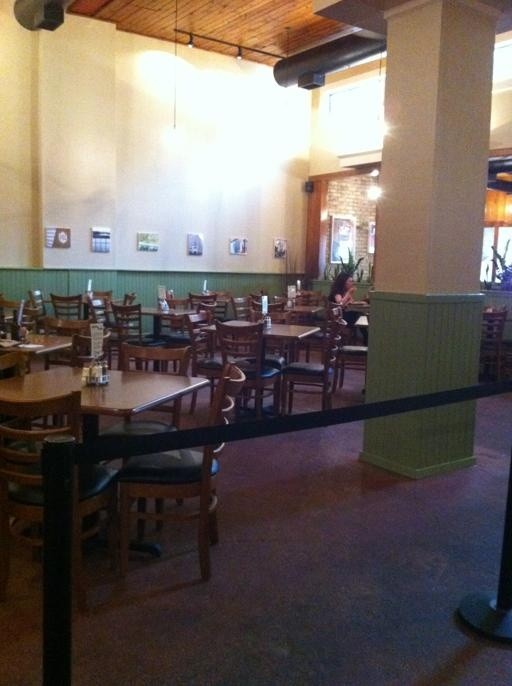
182 27 283 60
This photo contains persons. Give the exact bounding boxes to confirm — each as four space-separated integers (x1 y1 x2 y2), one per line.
329 271 368 340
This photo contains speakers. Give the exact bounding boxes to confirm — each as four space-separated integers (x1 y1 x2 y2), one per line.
305 181 314 193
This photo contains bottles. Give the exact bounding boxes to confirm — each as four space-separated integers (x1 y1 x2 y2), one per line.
256 313 271 329
82 361 110 387
0 307 20 341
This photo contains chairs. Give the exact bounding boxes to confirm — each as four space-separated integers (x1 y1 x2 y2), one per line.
481 305 509 384
0 288 368 584
